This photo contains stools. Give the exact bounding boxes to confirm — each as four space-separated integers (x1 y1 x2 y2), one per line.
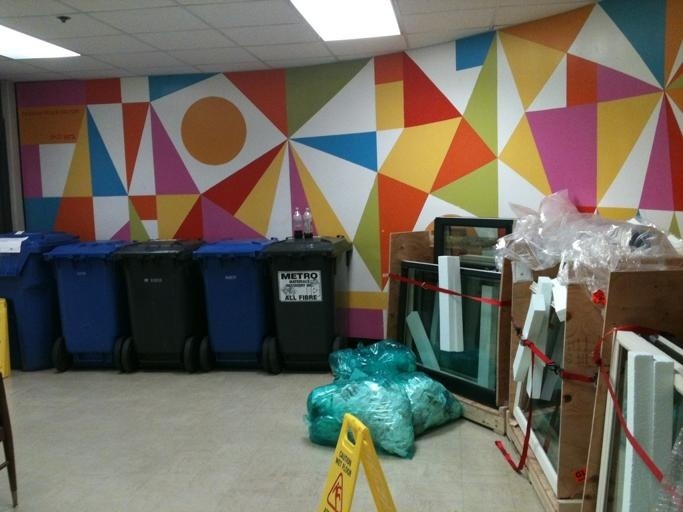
0 368 17 506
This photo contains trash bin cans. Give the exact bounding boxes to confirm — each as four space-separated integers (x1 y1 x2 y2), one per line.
191 232 274 370
1 233 66 371
110 232 202 371
47 234 123 370
258 232 353 374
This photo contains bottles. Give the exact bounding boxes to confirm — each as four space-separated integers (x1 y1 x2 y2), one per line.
301 208 313 242
292 207 304 239
